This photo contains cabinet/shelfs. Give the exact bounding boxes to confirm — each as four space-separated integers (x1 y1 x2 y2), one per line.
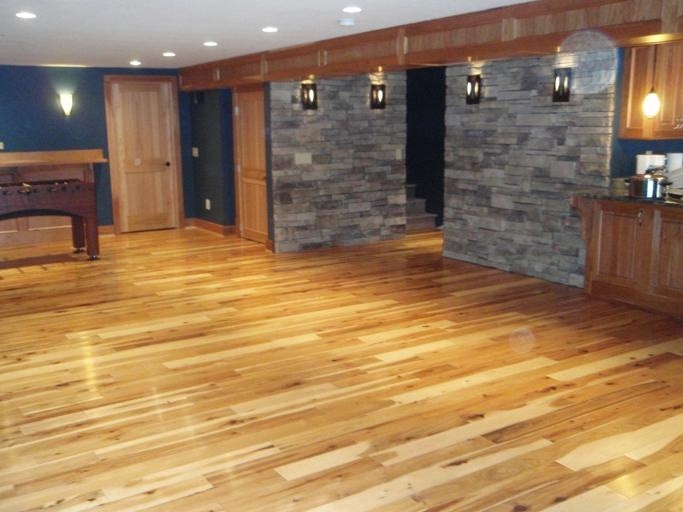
582 197 683 318
615 41 683 141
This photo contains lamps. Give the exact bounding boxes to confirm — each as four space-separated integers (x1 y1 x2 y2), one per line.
301 83 317 111
551 67 570 102
369 84 386 110
641 44 660 121
465 74 480 106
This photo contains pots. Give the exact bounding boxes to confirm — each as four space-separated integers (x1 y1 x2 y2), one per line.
623 169 674 200
